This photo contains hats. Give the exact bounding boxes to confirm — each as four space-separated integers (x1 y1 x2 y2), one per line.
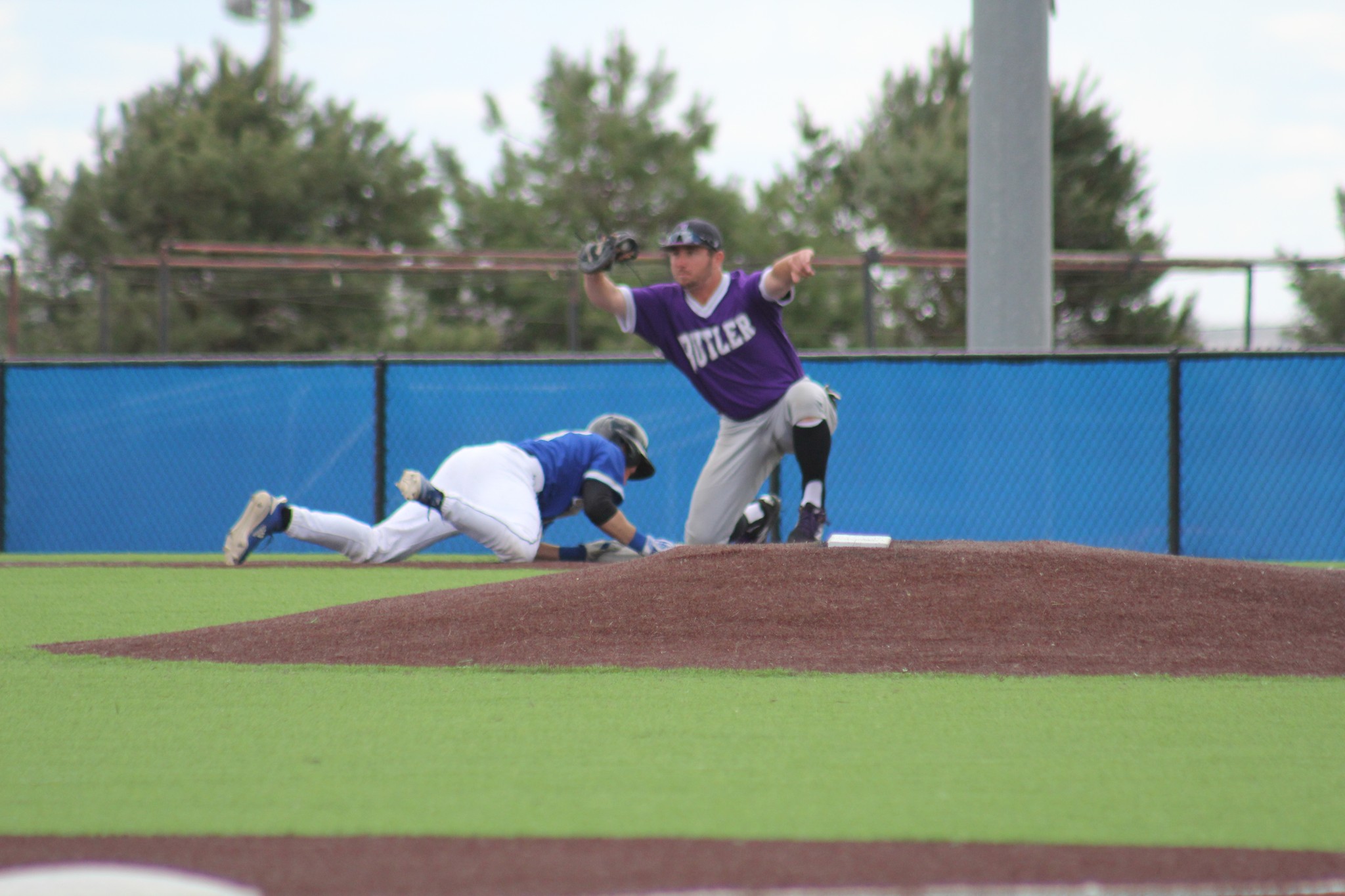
659 220 722 252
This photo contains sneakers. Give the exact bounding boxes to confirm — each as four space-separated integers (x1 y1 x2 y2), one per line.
736 494 780 544
395 469 444 516
787 502 830 544
223 490 292 567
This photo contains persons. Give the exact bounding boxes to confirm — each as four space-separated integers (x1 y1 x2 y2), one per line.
223 413 674 567
582 220 838 545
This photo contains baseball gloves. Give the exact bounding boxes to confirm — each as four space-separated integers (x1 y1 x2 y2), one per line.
577 232 639 275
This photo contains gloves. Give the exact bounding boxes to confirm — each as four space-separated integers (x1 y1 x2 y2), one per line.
627 528 674 557
558 540 621 562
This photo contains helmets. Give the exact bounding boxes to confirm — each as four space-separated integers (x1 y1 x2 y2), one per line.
587 416 655 481
